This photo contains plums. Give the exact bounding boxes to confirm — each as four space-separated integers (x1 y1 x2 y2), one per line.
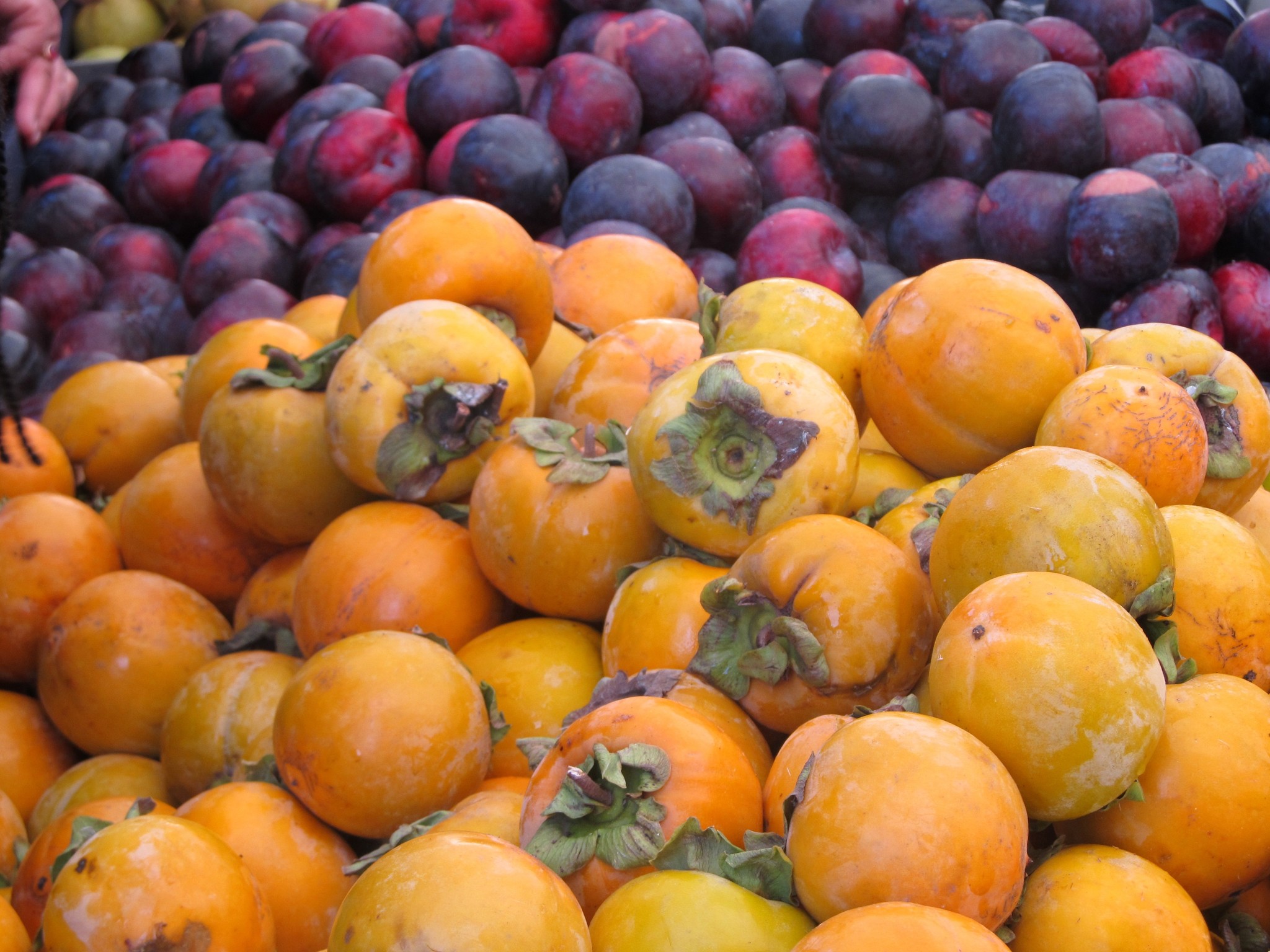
0 0 1267 406
